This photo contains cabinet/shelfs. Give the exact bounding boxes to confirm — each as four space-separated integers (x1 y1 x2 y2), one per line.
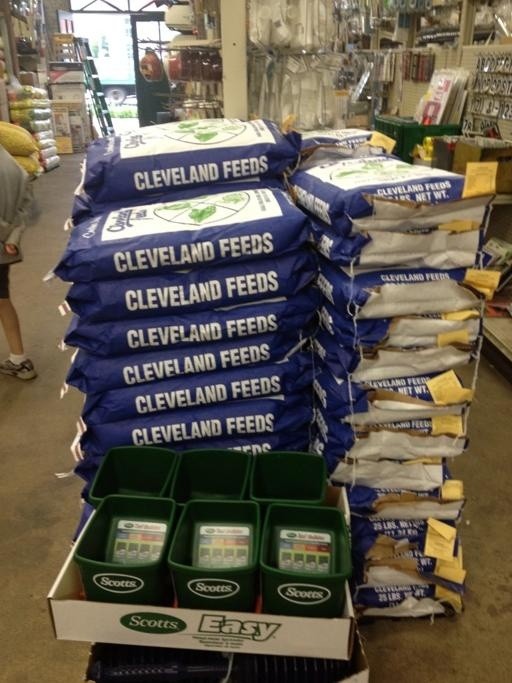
160 1 223 120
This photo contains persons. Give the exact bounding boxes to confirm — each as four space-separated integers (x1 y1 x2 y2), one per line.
1 142 38 380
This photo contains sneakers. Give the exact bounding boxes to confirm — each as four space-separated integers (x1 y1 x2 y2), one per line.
0 358 36 380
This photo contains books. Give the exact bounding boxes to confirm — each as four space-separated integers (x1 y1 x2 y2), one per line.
480 235 512 293
417 68 472 129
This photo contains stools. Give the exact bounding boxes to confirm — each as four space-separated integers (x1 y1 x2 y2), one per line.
52 32 79 63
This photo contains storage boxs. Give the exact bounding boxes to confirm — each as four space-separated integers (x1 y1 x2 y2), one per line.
83 621 373 682
454 138 512 195
375 115 461 164
45 507 359 663
